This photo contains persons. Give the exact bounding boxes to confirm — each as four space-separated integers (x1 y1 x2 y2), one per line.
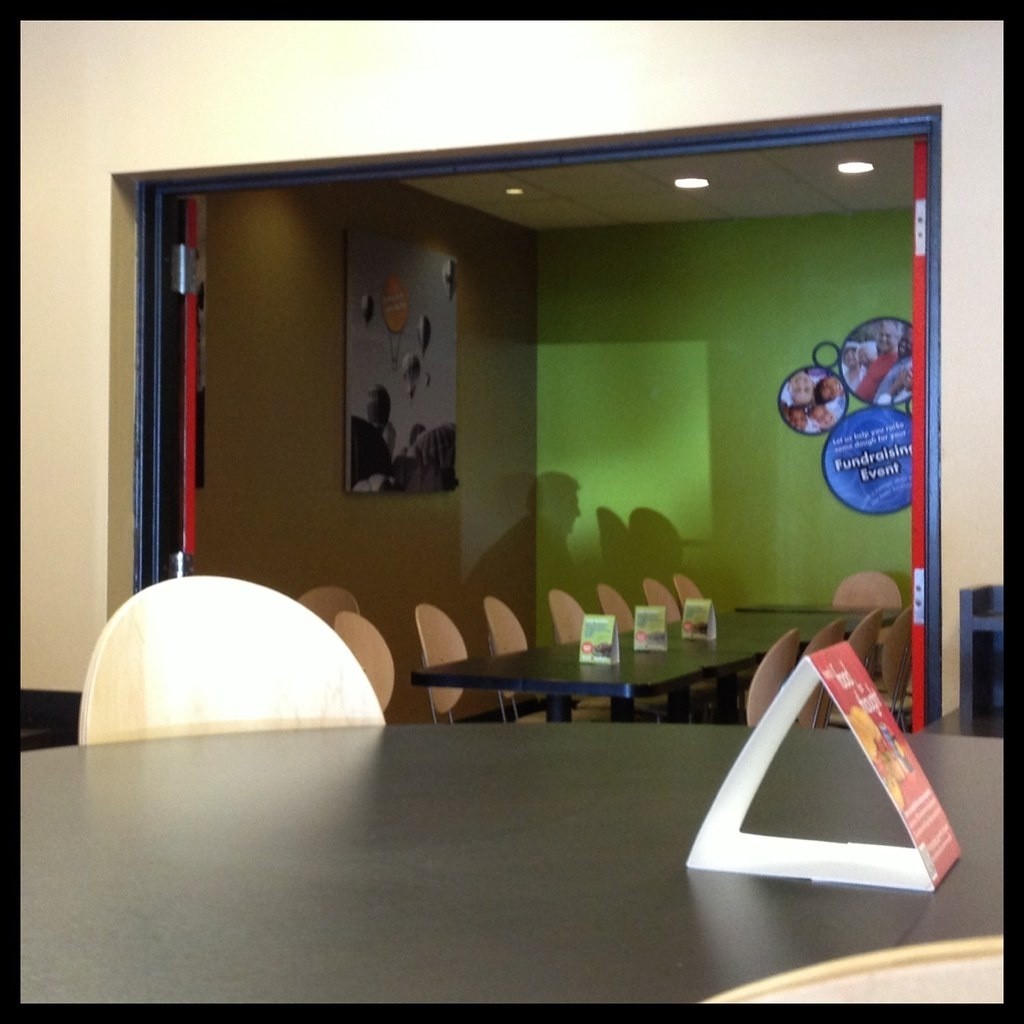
392 447 412 492
842 320 914 406
780 367 845 435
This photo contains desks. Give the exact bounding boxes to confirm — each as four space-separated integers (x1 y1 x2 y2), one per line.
21 722 1003 1003
410 604 906 725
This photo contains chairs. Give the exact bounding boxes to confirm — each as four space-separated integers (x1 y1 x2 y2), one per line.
335 611 394 712
78 575 386 744
746 572 912 732
549 572 717 724
296 587 359 630
415 604 469 723
484 597 611 722
